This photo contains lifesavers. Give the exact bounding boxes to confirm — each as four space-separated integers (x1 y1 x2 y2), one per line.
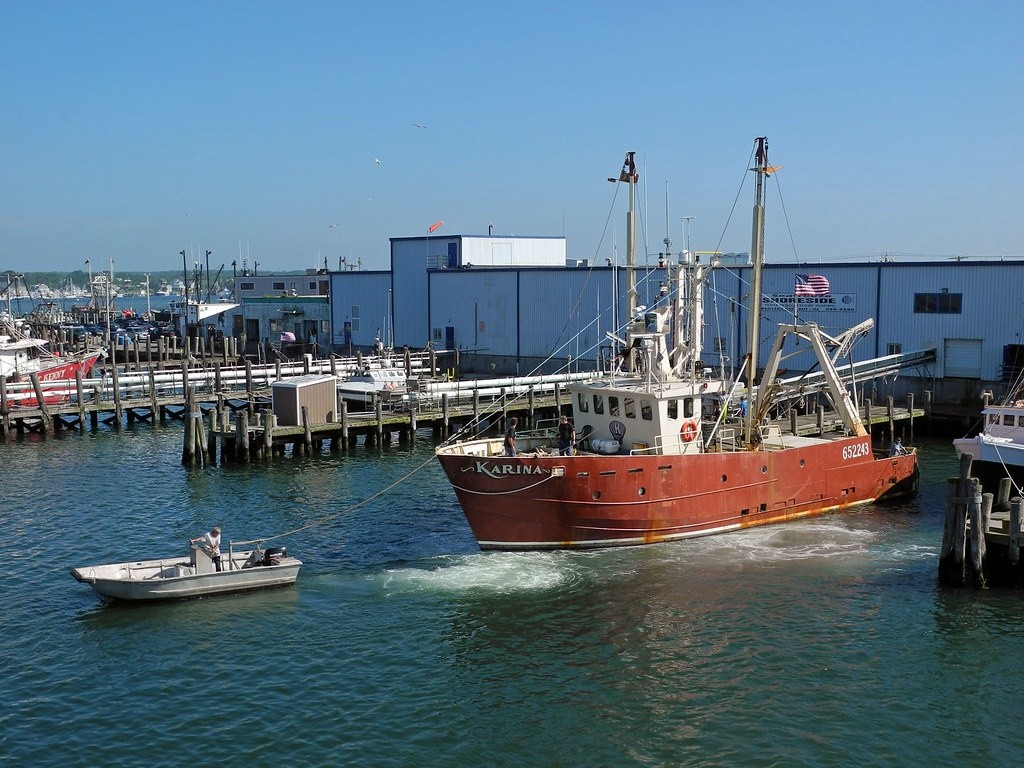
680 421 698 442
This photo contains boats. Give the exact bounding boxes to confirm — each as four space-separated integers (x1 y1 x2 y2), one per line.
434 136 922 551
68 537 303 602
0 272 101 417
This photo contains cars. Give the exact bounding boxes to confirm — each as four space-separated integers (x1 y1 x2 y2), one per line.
71 316 176 349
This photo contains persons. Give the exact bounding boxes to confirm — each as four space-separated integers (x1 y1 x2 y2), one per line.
503 417 519 458
192 527 221 571
557 416 576 455
217 311 227 327
376 338 383 353
737 396 748 417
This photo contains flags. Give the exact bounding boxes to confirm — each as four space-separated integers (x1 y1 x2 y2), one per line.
280 331 296 343
794 274 829 297
